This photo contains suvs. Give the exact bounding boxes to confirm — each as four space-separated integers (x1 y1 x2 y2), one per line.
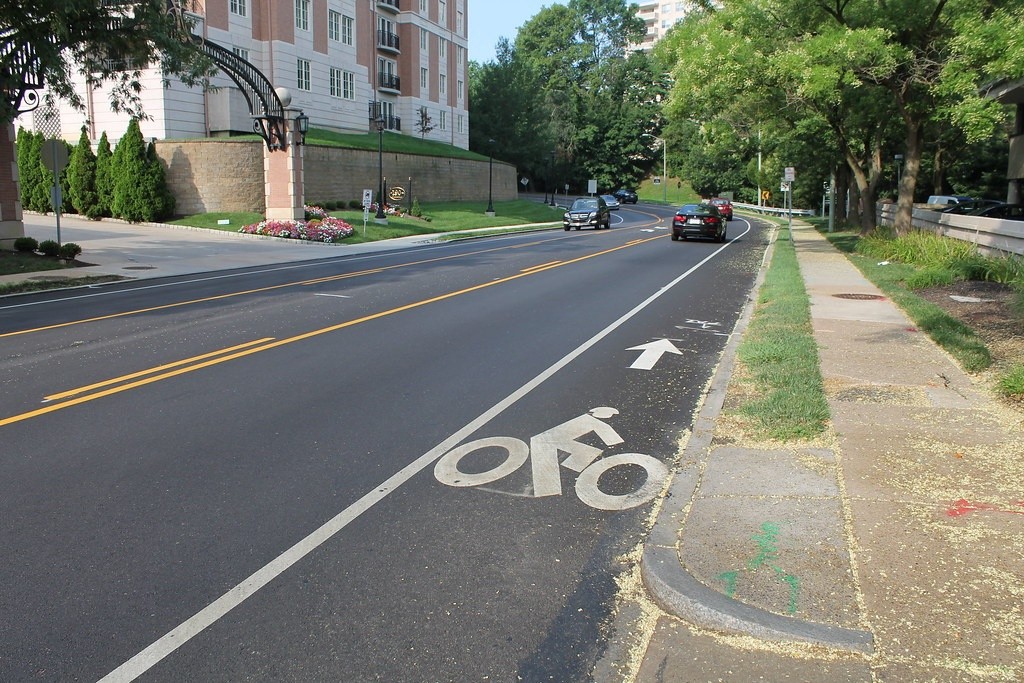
563 196 611 231
709 196 733 221
613 189 638 204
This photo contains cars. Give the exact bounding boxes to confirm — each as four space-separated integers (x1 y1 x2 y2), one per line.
599 194 620 211
672 203 728 243
927 196 1024 223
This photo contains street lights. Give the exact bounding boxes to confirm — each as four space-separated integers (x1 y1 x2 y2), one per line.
485 136 496 218
548 146 558 208
642 133 666 204
374 113 389 225
